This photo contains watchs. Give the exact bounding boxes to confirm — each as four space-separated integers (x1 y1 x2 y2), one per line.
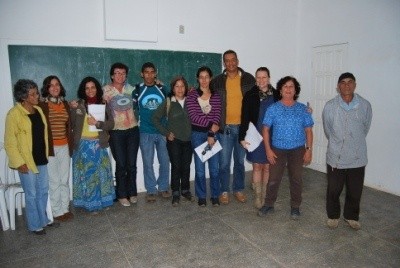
307 146 312 151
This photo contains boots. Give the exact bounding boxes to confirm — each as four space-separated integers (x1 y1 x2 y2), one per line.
255 190 267 208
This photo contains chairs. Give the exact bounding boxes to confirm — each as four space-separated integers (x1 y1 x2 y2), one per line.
0 147 54 231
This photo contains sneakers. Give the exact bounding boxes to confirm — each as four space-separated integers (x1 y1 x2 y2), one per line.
291 207 299 220
257 205 273 217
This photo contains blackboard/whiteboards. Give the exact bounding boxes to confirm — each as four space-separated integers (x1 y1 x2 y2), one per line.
8 45 222 101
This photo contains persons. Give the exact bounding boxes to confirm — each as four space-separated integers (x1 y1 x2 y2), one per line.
37 76 74 222
69 76 115 215
132 62 221 208
323 72 372 229
69 63 160 207
188 50 314 221
4 78 60 236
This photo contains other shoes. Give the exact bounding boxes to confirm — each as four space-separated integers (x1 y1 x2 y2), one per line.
119 198 132 207
327 216 340 229
32 229 46 234
56 212 74 221
159 191 171 199
181 190 194 201
344 218 360 229
90 206 112 215
172 190 180 201
209 196 220 206
129 196 137 203
147 193 156 202
198 197 206 207
233 191 247 202
47 222 60 228
220 191 229 203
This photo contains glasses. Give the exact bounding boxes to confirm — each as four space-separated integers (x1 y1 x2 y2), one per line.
114 71 126 77
